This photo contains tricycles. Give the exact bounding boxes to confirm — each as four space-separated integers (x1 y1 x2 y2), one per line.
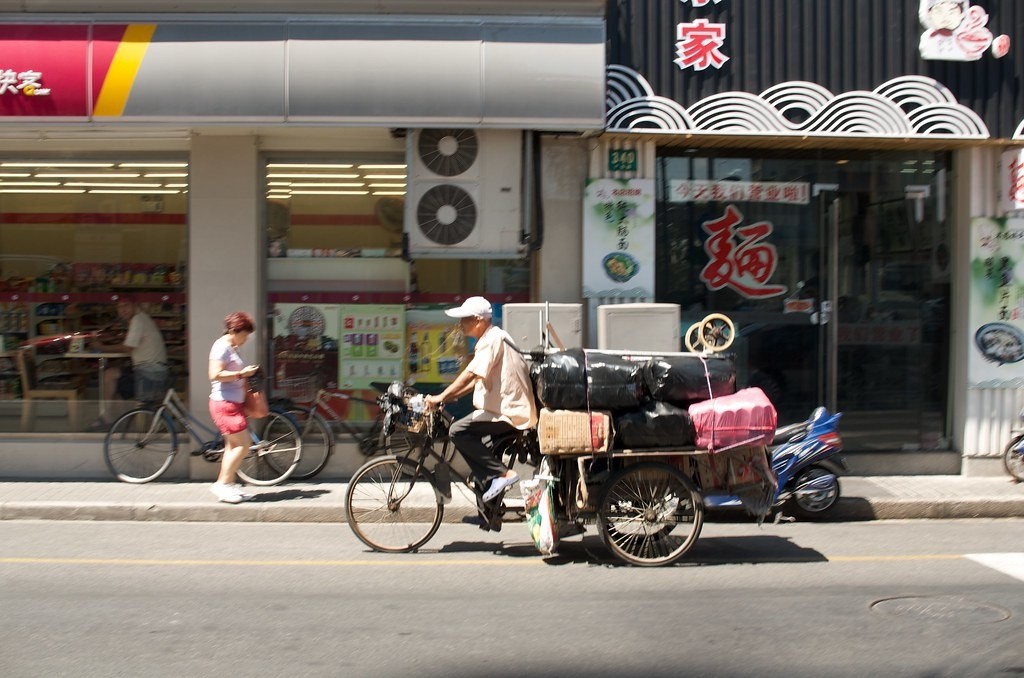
344 383 774 569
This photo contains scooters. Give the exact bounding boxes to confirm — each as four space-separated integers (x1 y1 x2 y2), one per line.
678 404 846 522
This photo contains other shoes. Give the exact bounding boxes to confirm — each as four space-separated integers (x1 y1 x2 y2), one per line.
86 415 113 432
211 482 252 503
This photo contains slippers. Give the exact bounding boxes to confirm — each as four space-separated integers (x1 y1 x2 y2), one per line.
462 514 501 532
483 472 520 503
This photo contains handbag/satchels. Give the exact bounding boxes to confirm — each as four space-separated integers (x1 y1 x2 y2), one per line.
245 391 270 418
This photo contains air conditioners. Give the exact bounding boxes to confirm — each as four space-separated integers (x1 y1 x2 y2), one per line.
408 126 535 260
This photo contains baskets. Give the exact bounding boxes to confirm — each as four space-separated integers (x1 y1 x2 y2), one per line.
395 394 429 434
277 375 318 403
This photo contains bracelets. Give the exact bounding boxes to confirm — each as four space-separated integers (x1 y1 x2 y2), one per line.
235 371 240 382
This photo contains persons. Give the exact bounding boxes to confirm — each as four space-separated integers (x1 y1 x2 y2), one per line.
423 296 538 526
208 313 260 503
88 293 167 432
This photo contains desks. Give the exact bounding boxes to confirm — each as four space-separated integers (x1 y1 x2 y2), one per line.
64 352 131 432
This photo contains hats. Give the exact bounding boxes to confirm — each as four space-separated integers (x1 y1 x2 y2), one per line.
444 296 493 319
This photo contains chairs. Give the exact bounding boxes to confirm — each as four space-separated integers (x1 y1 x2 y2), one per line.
15 349 189 433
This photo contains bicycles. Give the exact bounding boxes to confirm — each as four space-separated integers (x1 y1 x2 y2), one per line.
1005 432 1024 481
259 366 454 482
101 359 305 487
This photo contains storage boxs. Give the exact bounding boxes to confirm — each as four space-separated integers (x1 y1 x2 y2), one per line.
535 404 616 455
